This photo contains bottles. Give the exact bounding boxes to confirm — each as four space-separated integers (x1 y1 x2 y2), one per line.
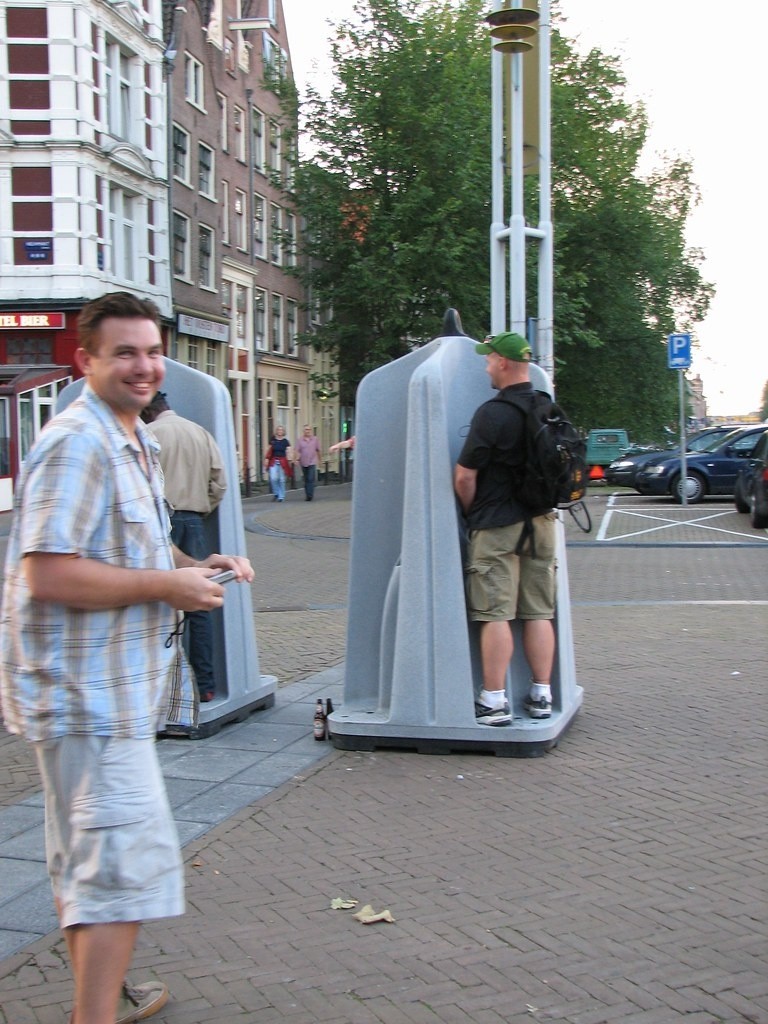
326 698 334 740
314 698 327 741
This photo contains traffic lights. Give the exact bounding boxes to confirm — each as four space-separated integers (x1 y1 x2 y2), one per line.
342 418 349 434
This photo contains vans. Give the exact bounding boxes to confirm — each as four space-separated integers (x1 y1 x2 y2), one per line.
585 429 630 467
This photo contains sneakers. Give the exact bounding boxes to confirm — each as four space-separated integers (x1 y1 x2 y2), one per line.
522 691 552 718
113 978 169 1024
474 698 513 726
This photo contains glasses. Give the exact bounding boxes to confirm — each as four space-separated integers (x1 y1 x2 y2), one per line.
483 334 503 358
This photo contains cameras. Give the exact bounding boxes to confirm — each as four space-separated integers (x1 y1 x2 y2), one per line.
209 569 237 585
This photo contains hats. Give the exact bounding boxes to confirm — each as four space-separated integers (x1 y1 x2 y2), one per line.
475 332 532 361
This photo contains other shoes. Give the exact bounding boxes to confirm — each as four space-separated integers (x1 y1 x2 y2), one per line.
272 495 278 501
201 692 213 701
305 497 312 501
278 499 283 502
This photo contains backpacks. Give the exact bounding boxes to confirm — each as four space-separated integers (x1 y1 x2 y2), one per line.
489 390 587 503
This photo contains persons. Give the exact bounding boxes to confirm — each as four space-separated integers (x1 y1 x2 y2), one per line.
0 291 254 1023
453 332 558 726
264 424 356 502
139 391 228 701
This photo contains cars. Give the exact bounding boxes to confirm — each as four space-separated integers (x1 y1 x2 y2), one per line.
734 428 768 529
604 423 764 495
631 426 768 505
